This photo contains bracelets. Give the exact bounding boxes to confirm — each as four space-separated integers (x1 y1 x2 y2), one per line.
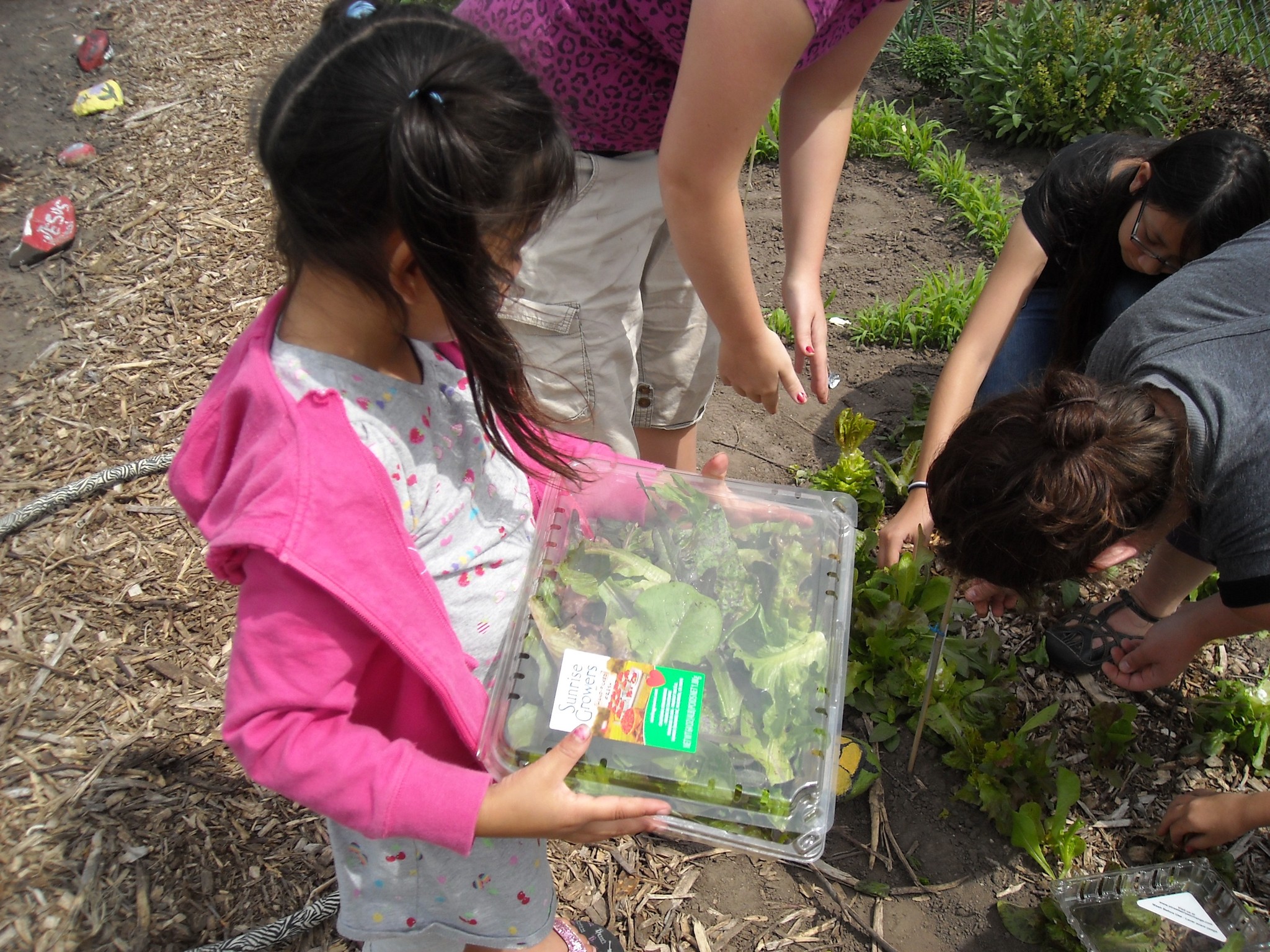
905 479 930 494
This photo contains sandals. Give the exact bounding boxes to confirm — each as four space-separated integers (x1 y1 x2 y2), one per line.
1045 589 1164 673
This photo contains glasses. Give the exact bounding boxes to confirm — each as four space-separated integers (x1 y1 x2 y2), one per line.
1128 180 1181 274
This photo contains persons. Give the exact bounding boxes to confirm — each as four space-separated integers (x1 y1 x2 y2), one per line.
432 0 908 478
166 7 821 952
925 208 1270 692
877 124 1270 586
1158 786 1270 857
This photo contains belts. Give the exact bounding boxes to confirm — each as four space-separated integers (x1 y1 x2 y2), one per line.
579 149 629 158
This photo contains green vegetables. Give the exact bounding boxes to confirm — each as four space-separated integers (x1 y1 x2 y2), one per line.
503 469 832 849
